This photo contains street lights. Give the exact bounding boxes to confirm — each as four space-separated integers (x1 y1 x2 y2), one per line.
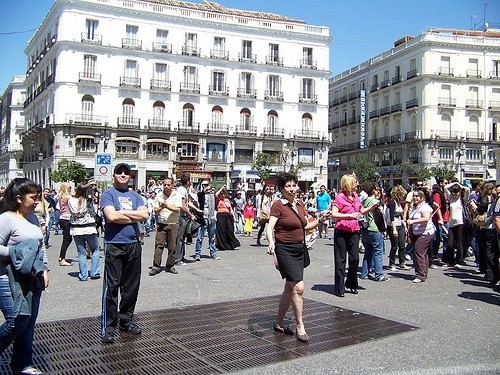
335 158 340 193
38 152 43 185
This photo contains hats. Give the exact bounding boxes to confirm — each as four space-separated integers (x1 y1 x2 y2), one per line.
113 163 132 175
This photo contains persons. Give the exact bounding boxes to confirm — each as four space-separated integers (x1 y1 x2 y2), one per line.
0 163 500 343
0 178 50 375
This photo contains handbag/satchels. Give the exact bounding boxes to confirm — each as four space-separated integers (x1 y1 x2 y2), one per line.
302 243 310 268
69 212 96 226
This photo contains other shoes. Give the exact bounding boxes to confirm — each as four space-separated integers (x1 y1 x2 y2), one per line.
350 288 358 294
405 254 411 261
335 291 344 297
21 368 43 375
211 254 221 260
100 332 114 343
175 260 187 266
119 324 141 335
378 276 390 281
59 259 73 266
274 326 293 334
296 328 310 341
430 257 469 270
149 267 161 275
412 278 422 283
194 254 200 261
166 267 178 274
389 263 413 271
